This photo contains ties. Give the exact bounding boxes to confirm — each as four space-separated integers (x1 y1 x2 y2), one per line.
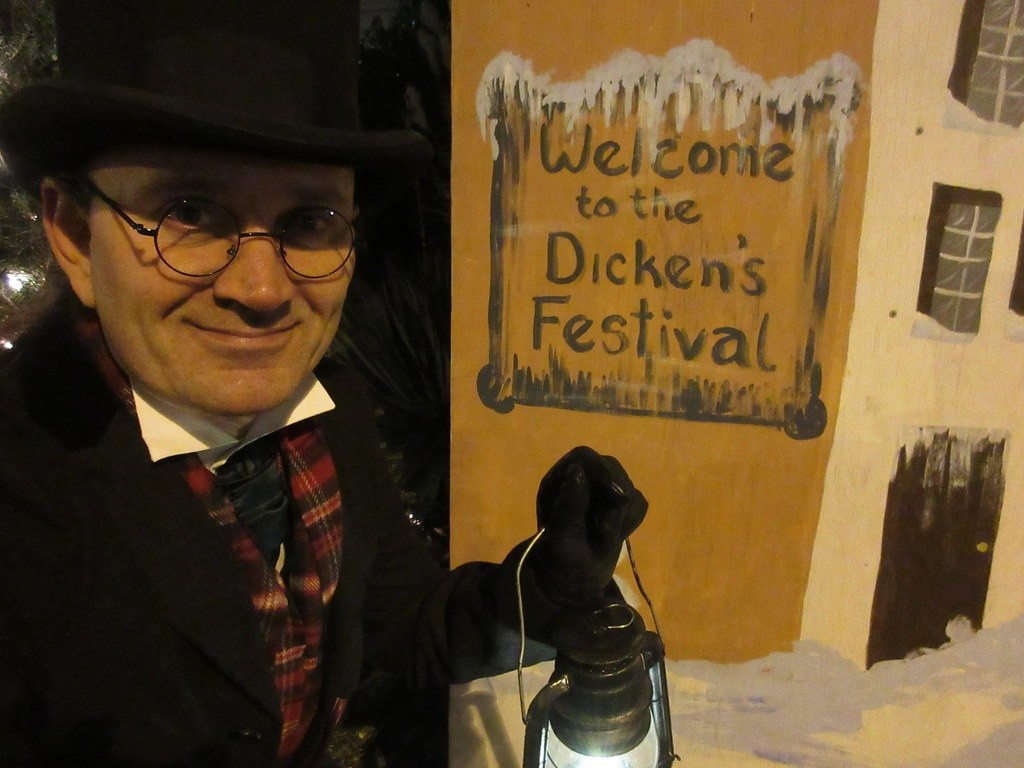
214 434 293 562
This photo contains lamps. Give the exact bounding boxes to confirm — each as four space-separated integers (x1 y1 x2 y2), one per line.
518 527 682 768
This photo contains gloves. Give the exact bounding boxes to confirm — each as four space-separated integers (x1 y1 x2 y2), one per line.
521 445 650 631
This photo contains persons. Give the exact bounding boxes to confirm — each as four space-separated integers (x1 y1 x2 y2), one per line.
0 0 649 768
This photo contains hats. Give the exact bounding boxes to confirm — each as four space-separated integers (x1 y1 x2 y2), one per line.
0 0 443 182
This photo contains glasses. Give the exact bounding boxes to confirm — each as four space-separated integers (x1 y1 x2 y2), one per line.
78 166 362 278
